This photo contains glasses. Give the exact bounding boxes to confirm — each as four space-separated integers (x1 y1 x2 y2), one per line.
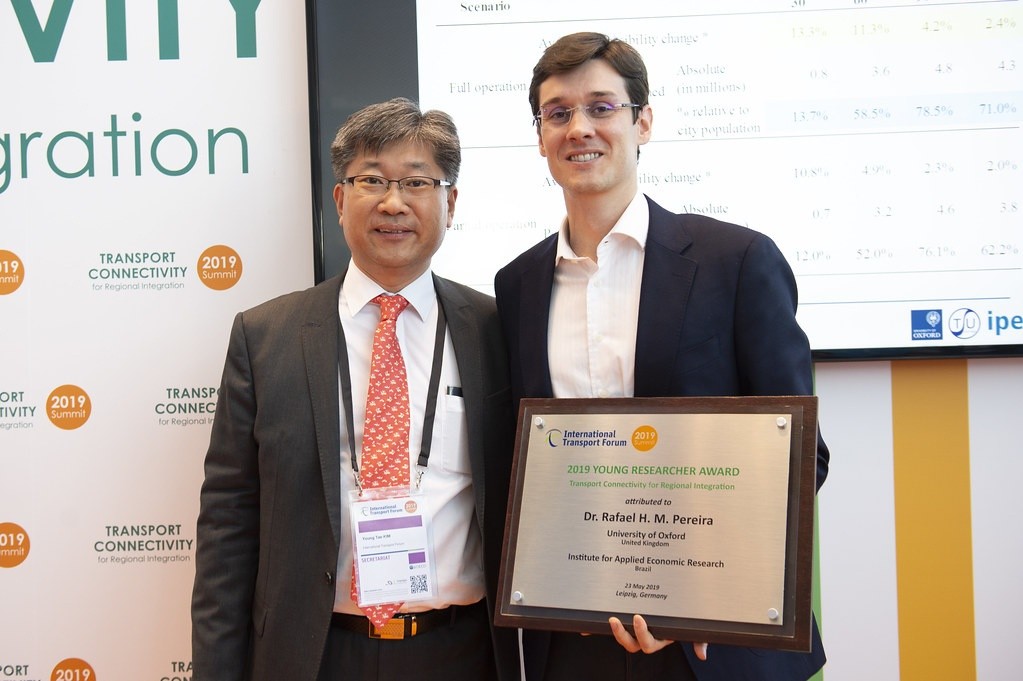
532 100 641 129
341 175 452 199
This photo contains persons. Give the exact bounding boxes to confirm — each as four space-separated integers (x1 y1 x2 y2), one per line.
191 99 505 681
493 32 830 681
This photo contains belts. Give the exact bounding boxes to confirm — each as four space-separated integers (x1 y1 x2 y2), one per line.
331 605 463 640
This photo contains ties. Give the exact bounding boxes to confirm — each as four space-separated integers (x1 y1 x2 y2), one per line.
350 295 410 632
